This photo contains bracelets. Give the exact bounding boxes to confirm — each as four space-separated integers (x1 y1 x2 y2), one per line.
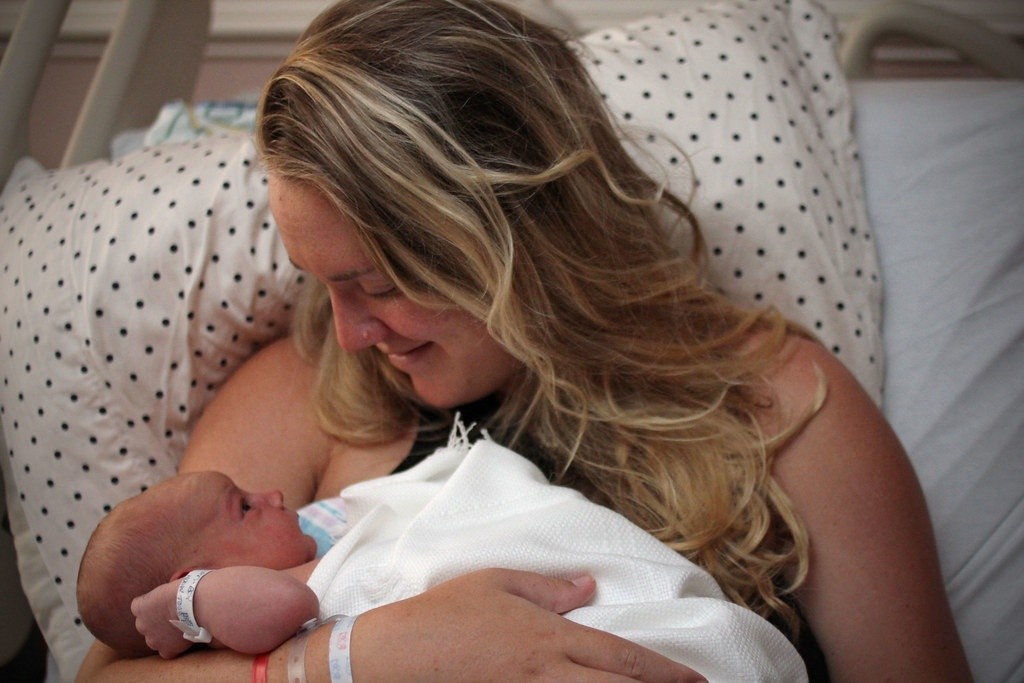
289 614 358 683
252 653 268 683
169 569 213 643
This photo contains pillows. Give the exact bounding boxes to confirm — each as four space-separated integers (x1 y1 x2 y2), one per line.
0 2 888 683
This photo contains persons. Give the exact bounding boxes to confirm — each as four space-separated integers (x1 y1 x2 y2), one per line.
75 437 809 683
74 0 975 683
